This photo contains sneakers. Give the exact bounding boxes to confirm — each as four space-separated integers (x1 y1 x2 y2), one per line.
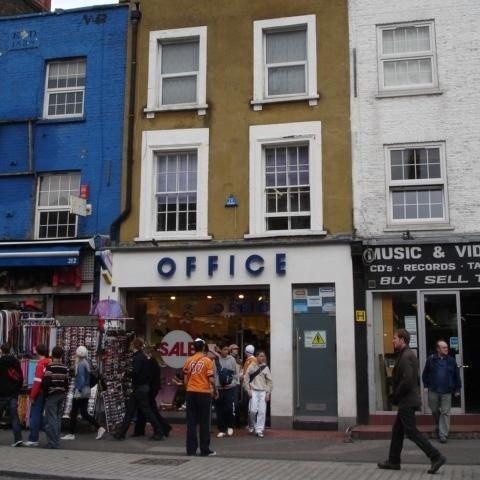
226 426 234 437
438 436 449 445
426 451 447 473
255 430 266 438
216 429 226 437
199 449 216 457
22 439 40 447
375 456 401 471
58 432 76 441
41 441 55 449
247 426 254 434
10 440 23 448
94 427 107 441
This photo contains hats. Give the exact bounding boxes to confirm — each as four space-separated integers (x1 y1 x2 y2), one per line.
244 344 255 354
75 345 89 357
192 337 204 342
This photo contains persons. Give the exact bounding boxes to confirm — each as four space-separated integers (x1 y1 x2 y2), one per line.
376 327 447 474
210 343 238 438
59 345 107 443
40 345 70 449
109 337 166 442
22 343 50 448
242 343 257 429
0 341 25 448
228 343 242 366
130 342 173 440
241 350 273 438
181 337 220 458
421 338 463 445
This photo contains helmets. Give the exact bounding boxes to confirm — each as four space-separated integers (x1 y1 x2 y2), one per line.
228 343 240 350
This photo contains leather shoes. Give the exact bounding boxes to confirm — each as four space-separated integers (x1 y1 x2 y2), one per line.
128 429 146 437
162 424 172 436
147 433 163 443
104 427 126 441
